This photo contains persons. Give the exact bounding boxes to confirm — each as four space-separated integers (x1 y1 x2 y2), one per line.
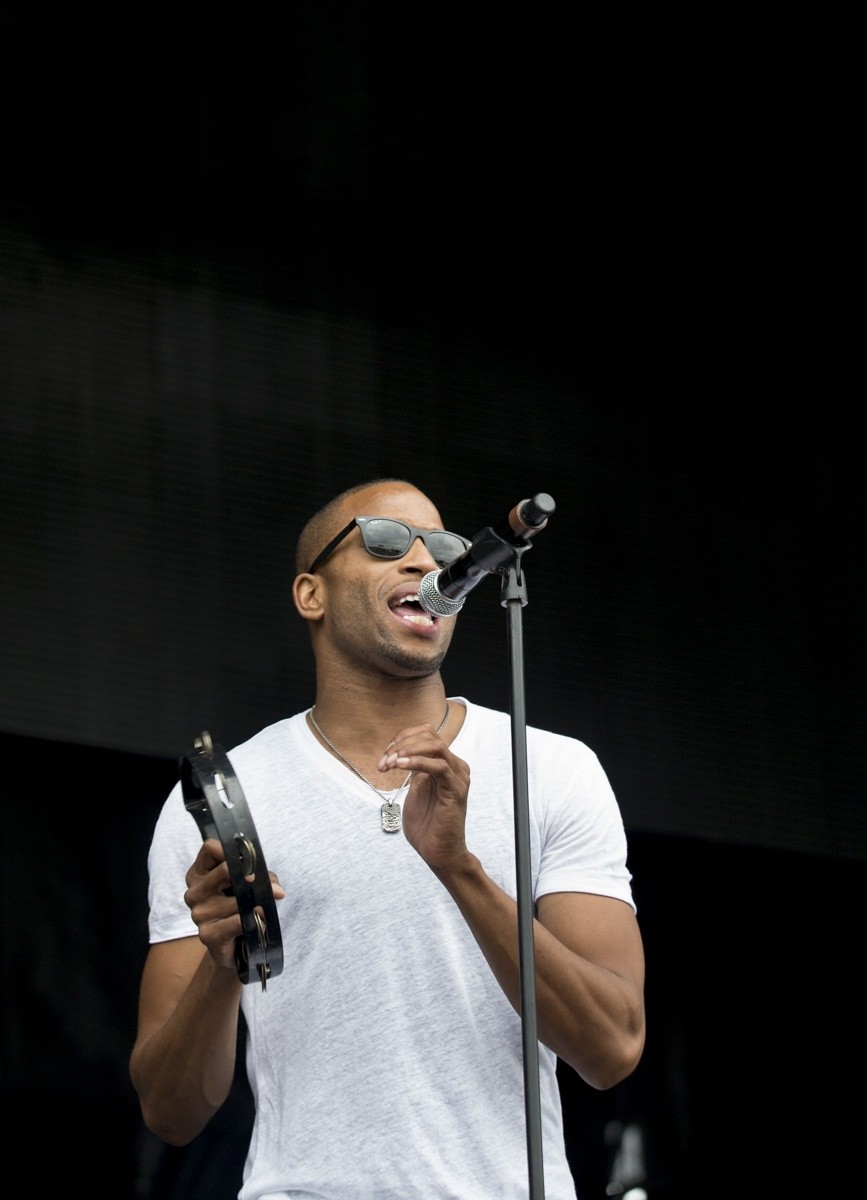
131 477 644 1198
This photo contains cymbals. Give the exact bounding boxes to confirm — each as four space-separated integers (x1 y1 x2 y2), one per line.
176 722 286 992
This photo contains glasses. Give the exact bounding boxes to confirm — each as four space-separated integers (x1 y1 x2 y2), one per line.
307 515 475 576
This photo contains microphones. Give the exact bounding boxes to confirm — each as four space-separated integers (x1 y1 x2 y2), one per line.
417 494 555 618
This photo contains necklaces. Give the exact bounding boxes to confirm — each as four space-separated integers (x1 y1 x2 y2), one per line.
308 700 449 834
